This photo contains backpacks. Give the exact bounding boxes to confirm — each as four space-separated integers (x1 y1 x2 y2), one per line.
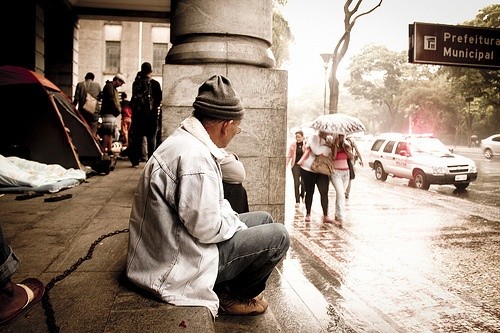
130 77 154 113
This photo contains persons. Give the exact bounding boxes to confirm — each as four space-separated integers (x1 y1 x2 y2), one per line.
127 61 163 168
345 136 363 199
120 92 133 146
122 74 292 317
297 131 334 224
471 138 476 148
72 72 102 134
396 142 411 157
286 131 307 208
100 73 126 159
0 226 46 326
330 134 353 229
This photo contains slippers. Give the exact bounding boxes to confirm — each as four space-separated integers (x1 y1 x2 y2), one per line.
44 193 73 201
0 278 45 326
15 190 50 200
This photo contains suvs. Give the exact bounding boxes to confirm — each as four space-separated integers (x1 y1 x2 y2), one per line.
367 133 478 192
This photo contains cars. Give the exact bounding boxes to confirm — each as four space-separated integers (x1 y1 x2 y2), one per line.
480 133 500 159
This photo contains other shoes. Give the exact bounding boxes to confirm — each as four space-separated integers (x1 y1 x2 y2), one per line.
217 293 269 315
108 151 114 157
323 216 333 223
305 214 311 221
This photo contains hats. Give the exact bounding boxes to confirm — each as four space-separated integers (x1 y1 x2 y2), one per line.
193 74 245 120
115 73 126 84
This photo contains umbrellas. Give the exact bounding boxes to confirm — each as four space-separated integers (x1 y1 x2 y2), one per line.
471 134 478 139
308 113 368 135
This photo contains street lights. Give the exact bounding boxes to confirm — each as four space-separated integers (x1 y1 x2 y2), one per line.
320 53 333 116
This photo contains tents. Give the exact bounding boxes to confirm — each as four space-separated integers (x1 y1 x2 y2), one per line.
0 63 111 175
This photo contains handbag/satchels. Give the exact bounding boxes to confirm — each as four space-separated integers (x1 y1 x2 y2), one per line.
311 153 335 176
82 92 98 115
347 156 355 179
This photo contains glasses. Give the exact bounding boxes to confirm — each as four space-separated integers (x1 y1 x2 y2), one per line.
218 118 242 135
